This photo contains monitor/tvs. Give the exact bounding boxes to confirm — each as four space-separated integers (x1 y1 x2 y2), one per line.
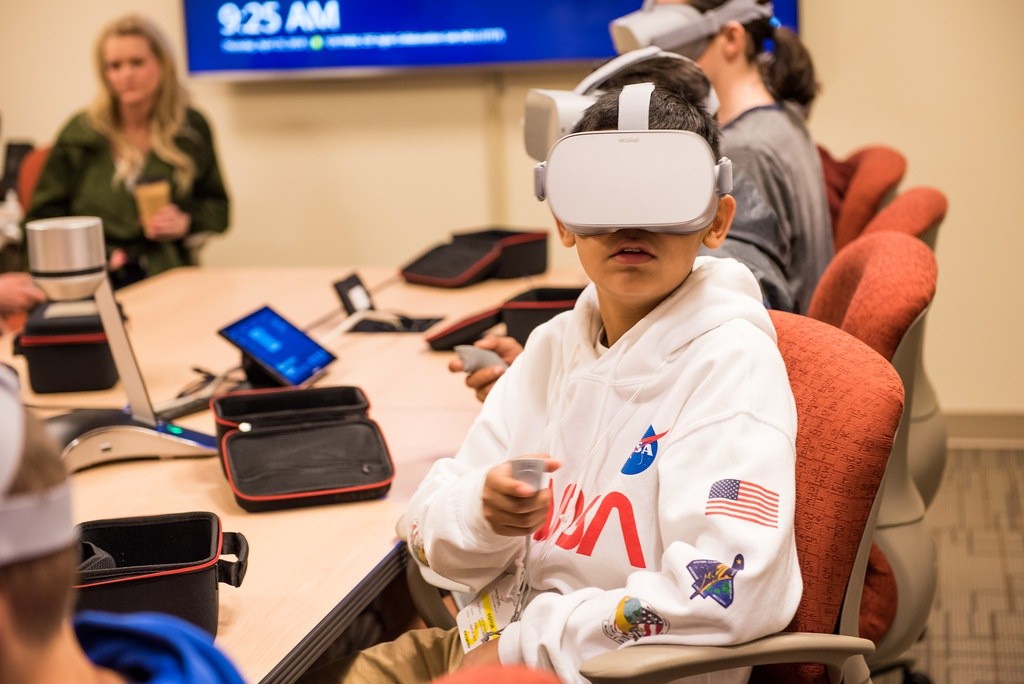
174 0 799 90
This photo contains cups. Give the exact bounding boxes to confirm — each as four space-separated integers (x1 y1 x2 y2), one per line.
132 173 171 239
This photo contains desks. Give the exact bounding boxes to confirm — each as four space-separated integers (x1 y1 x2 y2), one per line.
1 262 582 684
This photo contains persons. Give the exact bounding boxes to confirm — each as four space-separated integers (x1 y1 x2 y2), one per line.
0 272 45 312
296 81 803 684
446 46 803 406
609 0 834 309
16 12 231 288
0 408 247 684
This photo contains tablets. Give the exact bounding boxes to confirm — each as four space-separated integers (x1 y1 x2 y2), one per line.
216 304 338 388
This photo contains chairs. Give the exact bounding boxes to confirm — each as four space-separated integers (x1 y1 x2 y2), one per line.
401 139 955 684
